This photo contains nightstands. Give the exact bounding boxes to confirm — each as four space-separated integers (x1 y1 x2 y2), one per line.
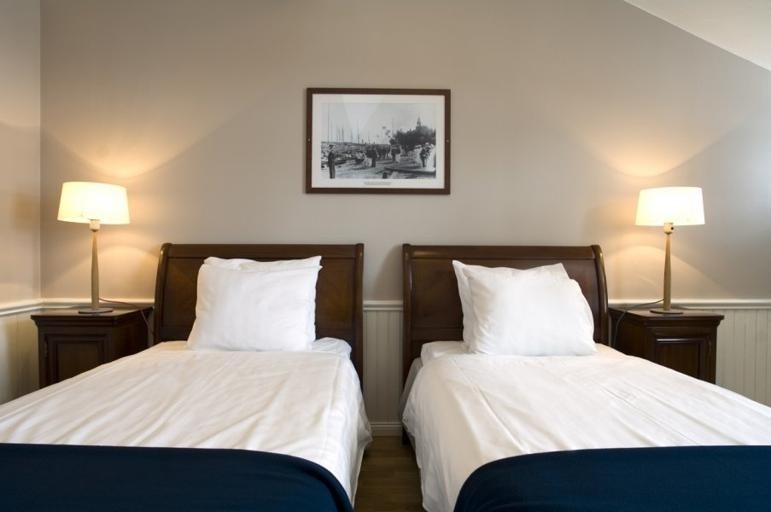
611 308 724 384
31 308 151 389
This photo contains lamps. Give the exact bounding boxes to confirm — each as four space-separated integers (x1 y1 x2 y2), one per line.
57 181 131 314
636 186 705 313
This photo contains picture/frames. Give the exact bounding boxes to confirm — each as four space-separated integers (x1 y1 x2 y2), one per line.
307 87 452 194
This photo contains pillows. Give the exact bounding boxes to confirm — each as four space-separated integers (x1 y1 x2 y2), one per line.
452 260 569 349
461 267 596 356
185 265 322 352
204 257 322 340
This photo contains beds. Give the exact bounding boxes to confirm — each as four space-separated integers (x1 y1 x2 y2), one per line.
403 243 771 511
0 242 372 511
